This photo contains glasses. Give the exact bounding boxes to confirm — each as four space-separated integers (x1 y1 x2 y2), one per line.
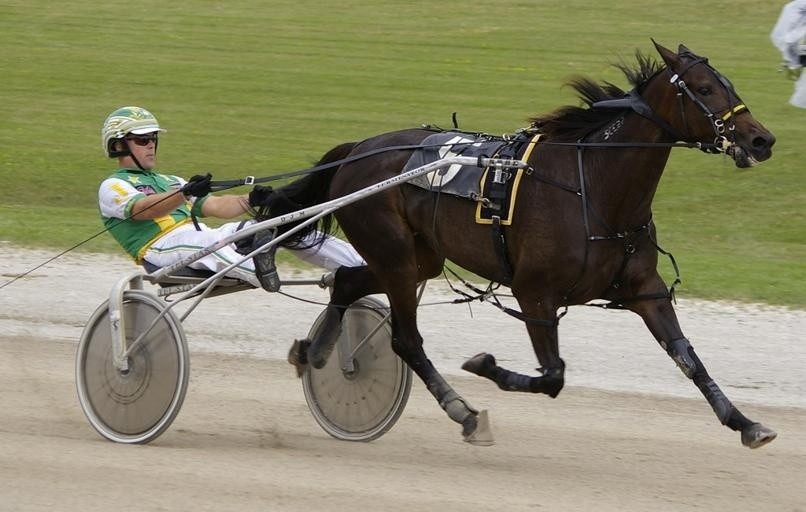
126 133 158 146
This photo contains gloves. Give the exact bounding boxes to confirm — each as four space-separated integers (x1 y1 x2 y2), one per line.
249 185 274 208
182 175 210 197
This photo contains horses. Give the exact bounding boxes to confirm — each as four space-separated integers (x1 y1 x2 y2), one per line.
235 34 779 450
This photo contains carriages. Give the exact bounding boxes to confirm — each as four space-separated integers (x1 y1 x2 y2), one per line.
75 37 777 447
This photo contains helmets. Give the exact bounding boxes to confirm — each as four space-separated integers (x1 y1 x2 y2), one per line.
102 106 167 159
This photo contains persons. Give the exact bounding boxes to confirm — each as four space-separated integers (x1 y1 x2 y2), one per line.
96 105 369 294
768 0 806 111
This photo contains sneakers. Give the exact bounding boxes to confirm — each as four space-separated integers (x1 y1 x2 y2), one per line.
251 229 281 291
